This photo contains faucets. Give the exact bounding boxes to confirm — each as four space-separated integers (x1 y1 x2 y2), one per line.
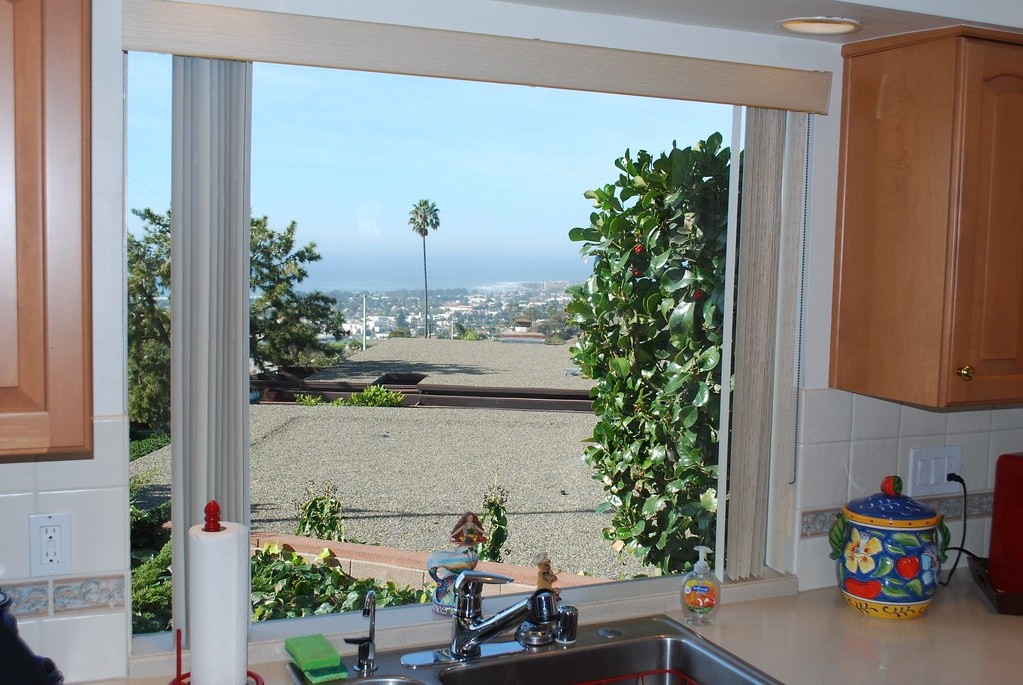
344 589 379 673
448 570 535 660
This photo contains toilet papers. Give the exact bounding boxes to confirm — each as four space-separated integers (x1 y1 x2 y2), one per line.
187 521 251 685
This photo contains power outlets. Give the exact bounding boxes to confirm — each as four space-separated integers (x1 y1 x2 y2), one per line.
28 513 73 575
908 442 962 498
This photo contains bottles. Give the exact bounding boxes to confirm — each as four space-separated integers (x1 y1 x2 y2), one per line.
828 475 950 619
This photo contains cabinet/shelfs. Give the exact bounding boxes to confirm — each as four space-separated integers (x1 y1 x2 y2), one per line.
0 0 96 464
828 25 1022 413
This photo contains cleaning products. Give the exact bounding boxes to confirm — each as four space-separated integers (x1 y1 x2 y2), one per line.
682 544 721 618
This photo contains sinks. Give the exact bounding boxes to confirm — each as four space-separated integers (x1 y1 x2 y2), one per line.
331 674 428 685
424 614 786 685
